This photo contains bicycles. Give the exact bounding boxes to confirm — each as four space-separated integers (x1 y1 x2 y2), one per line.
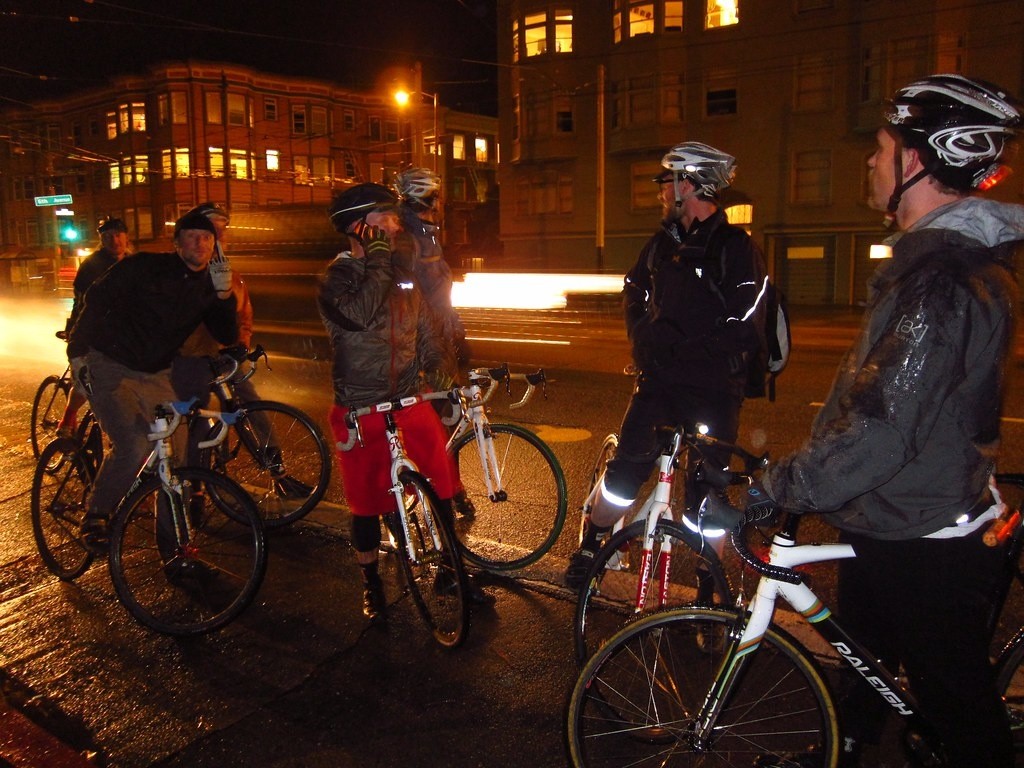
443 361 570 571
563 424 1024 768
30 328 334 529
334 382 474 650
578 364 773 746
30 396 270 636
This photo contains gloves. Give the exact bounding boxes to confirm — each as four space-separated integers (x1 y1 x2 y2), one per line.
654 217 688 256
424 367 453 393
208 240 232 291
360 225 392 258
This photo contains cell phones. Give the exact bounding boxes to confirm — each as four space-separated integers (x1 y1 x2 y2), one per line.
353 222 373 240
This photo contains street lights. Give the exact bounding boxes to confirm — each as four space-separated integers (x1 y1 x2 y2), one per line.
392 86 445 253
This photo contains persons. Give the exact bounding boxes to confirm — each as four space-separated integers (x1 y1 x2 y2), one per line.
172 202 315 525
399 165 476 518
59 216 131 433
67 216 238 576
317 183 496 621
566 142 772 656
742 72 1024 768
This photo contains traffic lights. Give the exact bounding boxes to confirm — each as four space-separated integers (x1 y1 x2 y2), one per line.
61 225 77 242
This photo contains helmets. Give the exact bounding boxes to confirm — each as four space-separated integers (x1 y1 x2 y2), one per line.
880 73 1024 191
392 167 441 202
188 201 231 225
652 142 739 199
326 183 398 233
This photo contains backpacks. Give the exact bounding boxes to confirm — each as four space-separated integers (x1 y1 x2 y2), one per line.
652 224 792 404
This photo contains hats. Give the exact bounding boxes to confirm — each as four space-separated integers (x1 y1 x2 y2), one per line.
96 215 127 234
174 213 217 239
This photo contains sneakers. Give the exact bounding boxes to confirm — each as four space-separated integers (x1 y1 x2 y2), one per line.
79 511 110 553
160 554 220 580
432 567 497 612
564 547 600 596
363 579 388 631
273 475 315 500
695 623 729 654
